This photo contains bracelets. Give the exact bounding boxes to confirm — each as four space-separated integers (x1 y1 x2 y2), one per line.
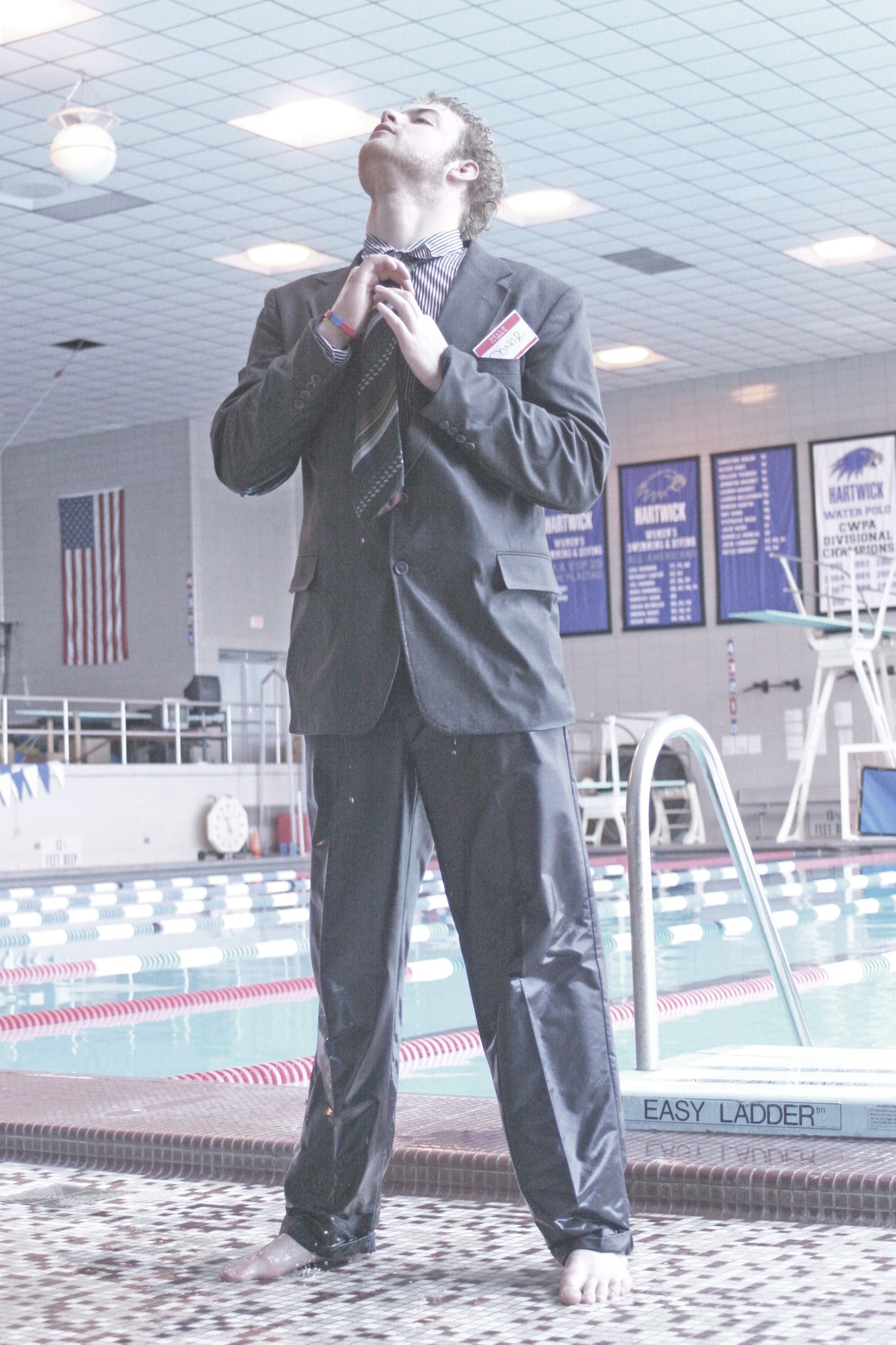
323 309 360 340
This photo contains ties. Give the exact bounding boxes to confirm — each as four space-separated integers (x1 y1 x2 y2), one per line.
346 253 410 535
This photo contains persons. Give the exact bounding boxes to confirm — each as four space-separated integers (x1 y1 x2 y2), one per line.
208 90 634 1306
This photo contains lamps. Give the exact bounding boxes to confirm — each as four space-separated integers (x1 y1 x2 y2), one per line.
51 67 115 186
